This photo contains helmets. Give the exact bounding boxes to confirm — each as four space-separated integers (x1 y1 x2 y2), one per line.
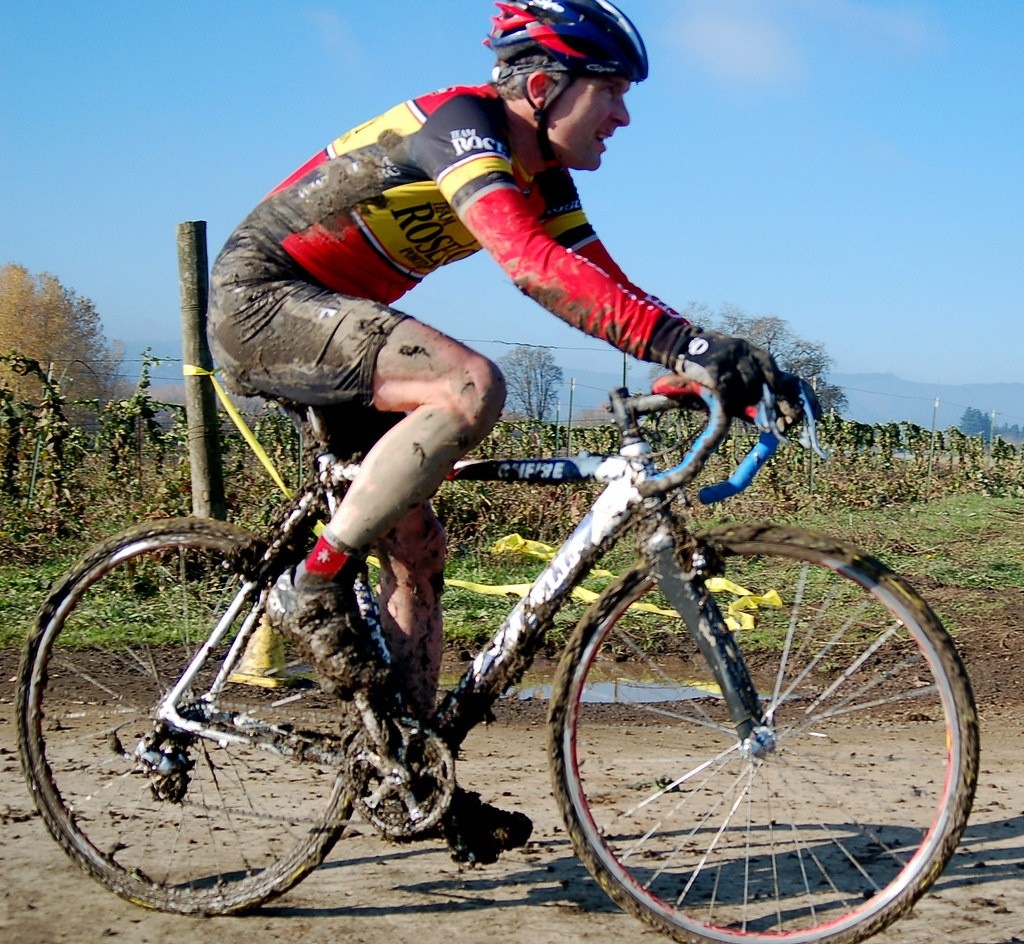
484 0 649 82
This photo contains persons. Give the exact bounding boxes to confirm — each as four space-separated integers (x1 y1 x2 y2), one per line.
207 0 805 852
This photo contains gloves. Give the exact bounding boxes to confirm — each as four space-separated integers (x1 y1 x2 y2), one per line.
727 384 803 435
666 323 779 400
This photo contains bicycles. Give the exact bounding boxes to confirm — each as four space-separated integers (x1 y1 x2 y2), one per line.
13 364 985 944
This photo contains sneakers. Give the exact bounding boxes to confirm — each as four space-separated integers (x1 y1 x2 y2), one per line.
264 565 390 697
378 776 533 852
